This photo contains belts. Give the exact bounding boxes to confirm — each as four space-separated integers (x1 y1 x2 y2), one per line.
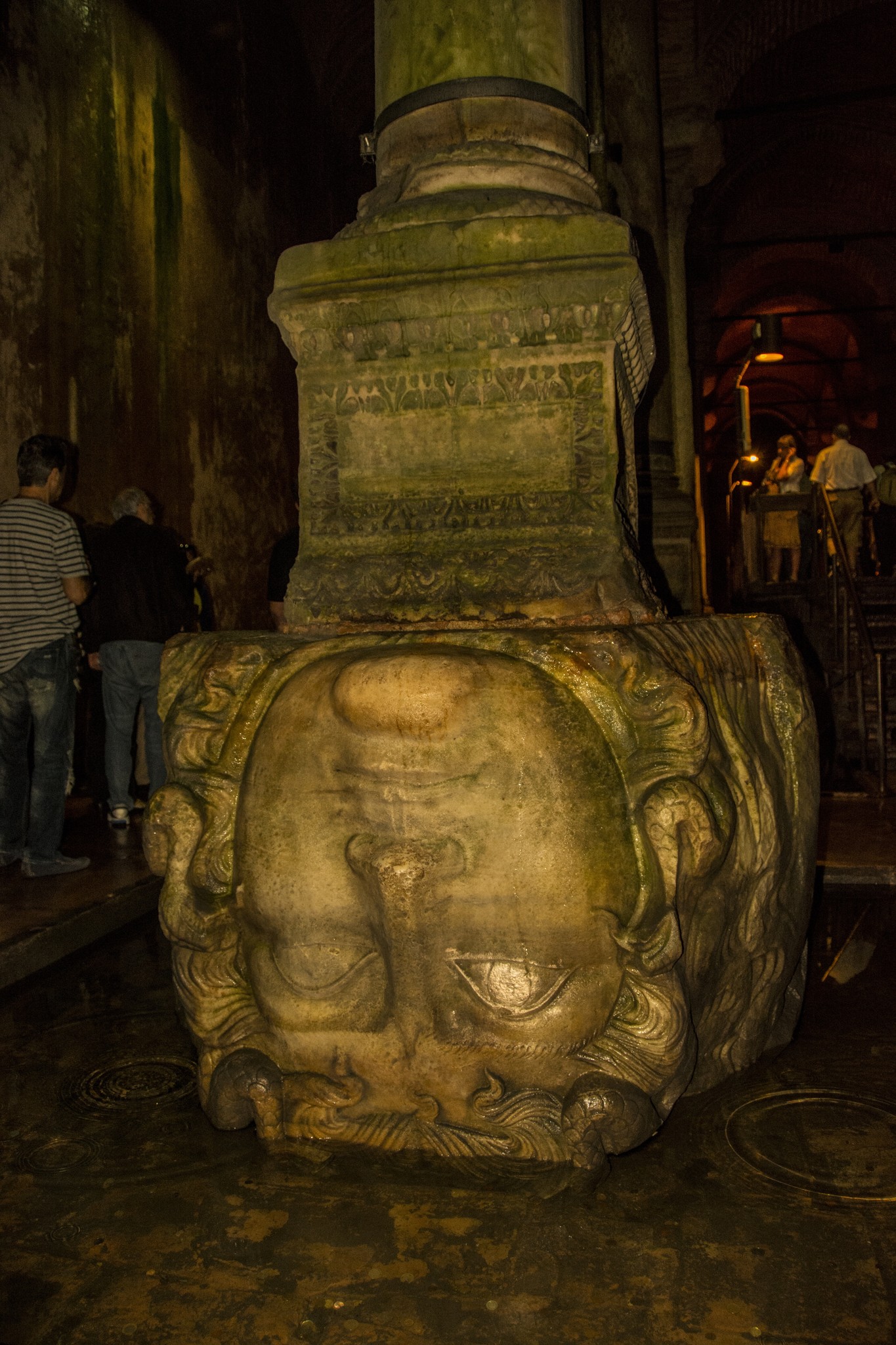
826 486 861 493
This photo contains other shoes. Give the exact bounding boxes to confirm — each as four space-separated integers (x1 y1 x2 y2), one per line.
829 563 842 577
767 576 778 584
852 571 859 577
789 574 798 583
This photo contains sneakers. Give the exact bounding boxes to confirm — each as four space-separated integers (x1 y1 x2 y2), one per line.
107 805 130 827
0 849 28 868
110 826 132 849
22 854 90 874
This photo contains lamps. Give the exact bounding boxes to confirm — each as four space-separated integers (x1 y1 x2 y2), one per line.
750 315 785 364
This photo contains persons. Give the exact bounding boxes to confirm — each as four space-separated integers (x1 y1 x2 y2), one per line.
138 607 823 1166
133 703 165 812
725 391 896 605
173 513 299 632
0 430 99 886
79 483 187 826
